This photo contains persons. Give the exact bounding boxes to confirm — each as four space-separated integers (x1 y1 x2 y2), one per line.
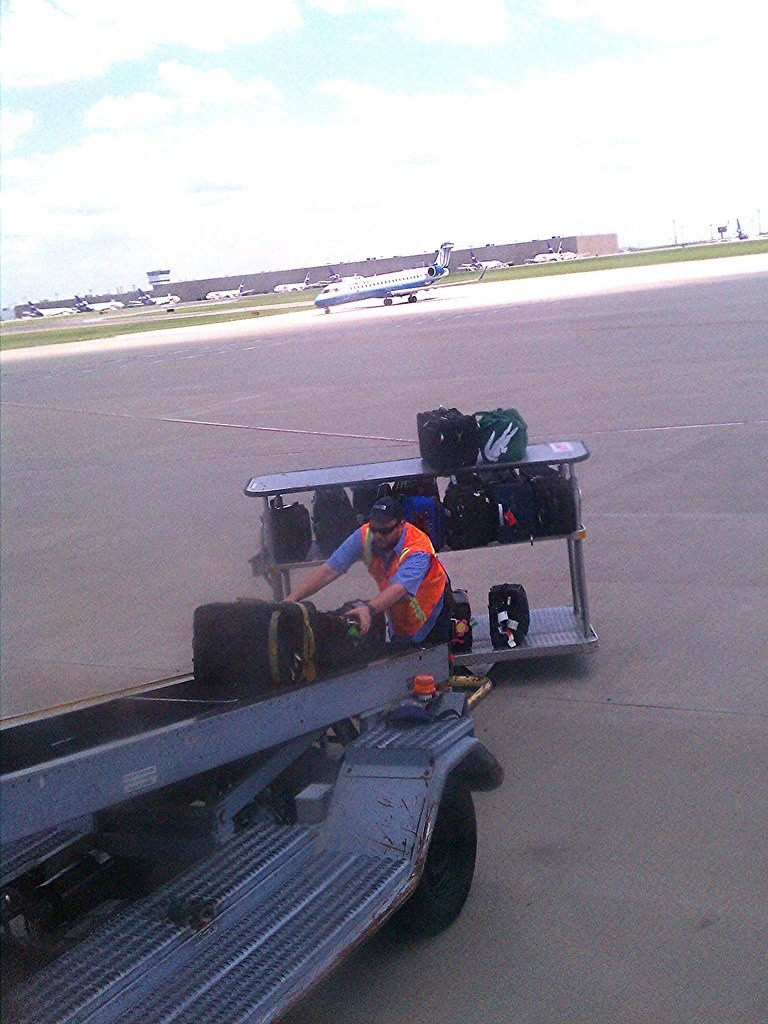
277 496 456 649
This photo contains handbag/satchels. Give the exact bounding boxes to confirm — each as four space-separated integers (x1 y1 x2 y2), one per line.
317 598 387 666
418 408 481 472
473 408 527 463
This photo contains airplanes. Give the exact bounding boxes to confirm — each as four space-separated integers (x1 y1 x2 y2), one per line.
525 241 576 265
313 242 454 316
205 279 254 300
457 249 509 273
22 301 74 318
273 272 319 293
72 294 124 313
137 287 180 307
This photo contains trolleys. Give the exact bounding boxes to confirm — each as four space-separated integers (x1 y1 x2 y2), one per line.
239 439 604 685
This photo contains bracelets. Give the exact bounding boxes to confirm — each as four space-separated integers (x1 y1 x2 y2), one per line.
287 586 303 604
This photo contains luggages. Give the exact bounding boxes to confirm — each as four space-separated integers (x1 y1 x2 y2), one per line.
447 588 472 651
192 601 321 680
268 466 575 560
488 583 529 647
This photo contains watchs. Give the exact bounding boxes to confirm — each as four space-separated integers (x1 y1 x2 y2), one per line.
362 602 378 621
